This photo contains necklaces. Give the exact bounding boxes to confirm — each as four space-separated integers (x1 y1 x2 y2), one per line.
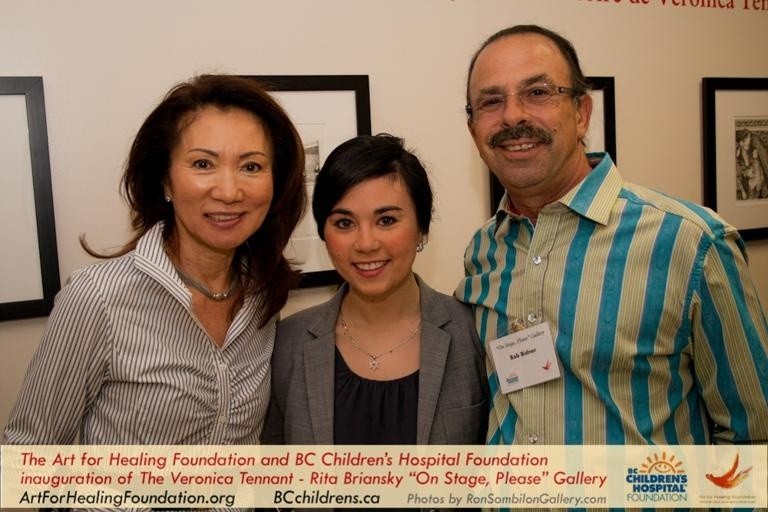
172 266 240 301
337 307 422 373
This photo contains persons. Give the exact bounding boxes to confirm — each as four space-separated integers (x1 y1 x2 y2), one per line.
736 129 766 200
260 131 490 512
451 24 768 512
0 73 308 511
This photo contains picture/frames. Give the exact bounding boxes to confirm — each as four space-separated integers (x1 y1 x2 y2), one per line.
0 76 768 325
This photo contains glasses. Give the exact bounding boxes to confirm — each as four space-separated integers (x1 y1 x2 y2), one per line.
477 82 583 113
